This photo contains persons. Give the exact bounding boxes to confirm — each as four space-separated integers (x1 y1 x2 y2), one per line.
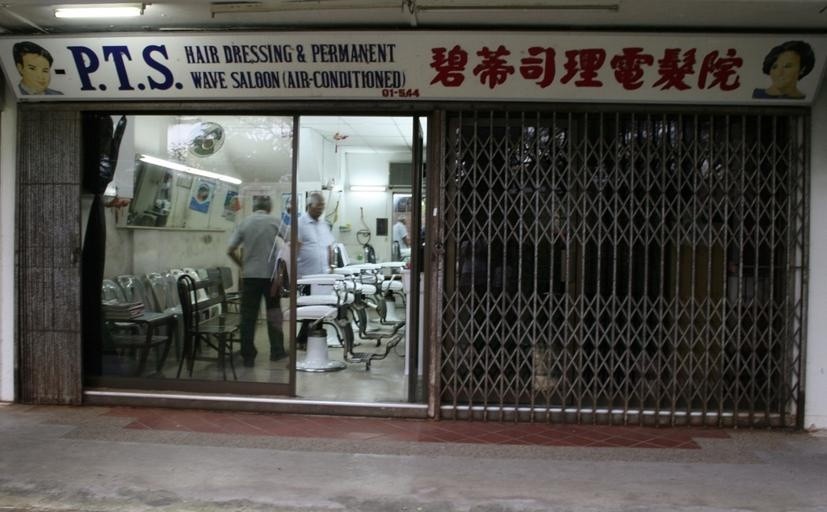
11 41 63 97
392 215 410 250
296 192 333 352
753 40 815 100
225 193 290 366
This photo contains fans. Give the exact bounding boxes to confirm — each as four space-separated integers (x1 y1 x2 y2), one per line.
170 120 224 164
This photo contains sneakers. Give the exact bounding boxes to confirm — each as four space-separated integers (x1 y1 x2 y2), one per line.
245 341 306 368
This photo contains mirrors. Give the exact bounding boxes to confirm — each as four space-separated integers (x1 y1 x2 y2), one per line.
391 189 411 261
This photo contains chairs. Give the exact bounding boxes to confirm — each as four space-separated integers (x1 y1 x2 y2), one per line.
100 241 408 380
